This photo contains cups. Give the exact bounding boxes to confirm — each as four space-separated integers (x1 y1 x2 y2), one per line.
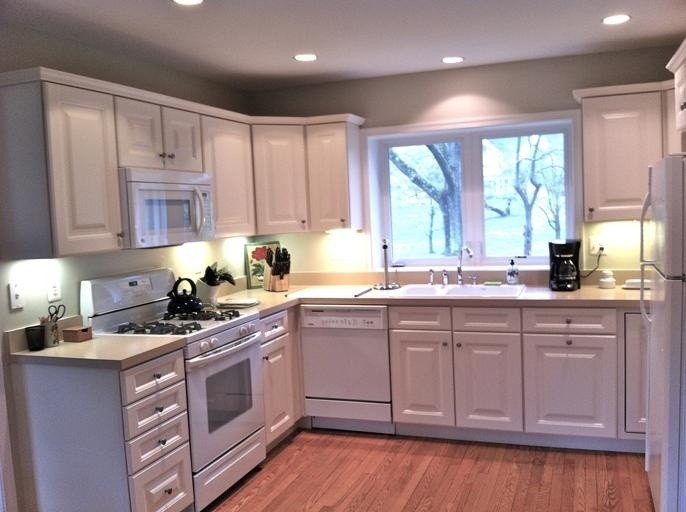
24 325 45 352
39 322 60 348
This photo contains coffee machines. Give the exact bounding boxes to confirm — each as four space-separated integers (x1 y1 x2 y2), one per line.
547 239 584 292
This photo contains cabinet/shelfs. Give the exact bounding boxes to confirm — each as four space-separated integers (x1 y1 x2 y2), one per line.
663 36 686 130
385 303 525 435
201 114 254 237
2 314 196 512
520 306 620 441
1 79 122 259
113 94 205 170
305 120 364 228
251 122 307 234
580 92 664 222
618 310 650 438
258 309 295 445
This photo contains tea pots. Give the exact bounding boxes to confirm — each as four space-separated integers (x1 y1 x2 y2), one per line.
164 276 204 314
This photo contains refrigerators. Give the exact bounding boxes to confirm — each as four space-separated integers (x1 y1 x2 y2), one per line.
636 153 686 511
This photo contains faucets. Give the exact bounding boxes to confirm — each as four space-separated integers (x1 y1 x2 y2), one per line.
441 270 449 285
457 246 473 285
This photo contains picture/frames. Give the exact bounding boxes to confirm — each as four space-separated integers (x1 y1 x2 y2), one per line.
243 241 281 291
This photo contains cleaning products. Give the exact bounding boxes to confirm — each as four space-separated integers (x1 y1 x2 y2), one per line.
506 259 520 286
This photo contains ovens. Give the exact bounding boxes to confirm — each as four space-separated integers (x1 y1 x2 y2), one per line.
184 332 268 511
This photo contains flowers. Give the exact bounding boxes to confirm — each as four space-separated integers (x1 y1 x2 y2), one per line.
250 246 266 277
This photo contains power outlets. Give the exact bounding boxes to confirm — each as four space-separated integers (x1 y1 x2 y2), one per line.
590 233 609 256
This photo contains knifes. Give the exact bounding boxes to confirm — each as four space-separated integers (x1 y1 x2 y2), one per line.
265 245 293 278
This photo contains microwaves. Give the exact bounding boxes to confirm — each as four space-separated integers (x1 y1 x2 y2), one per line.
117 164 220 247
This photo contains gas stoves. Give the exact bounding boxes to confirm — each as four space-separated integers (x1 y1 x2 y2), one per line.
111 305 262 359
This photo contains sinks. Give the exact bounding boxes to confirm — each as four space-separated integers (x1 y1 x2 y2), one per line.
403 283 449 297
445 285 523 299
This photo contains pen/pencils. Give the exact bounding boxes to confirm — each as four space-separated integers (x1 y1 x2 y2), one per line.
39 315 58 323
354 287 372 297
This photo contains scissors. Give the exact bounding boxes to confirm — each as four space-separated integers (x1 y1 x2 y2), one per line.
48 305 66 322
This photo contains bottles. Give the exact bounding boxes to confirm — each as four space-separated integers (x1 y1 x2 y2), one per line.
505 259 519 285
598 269 617 289
